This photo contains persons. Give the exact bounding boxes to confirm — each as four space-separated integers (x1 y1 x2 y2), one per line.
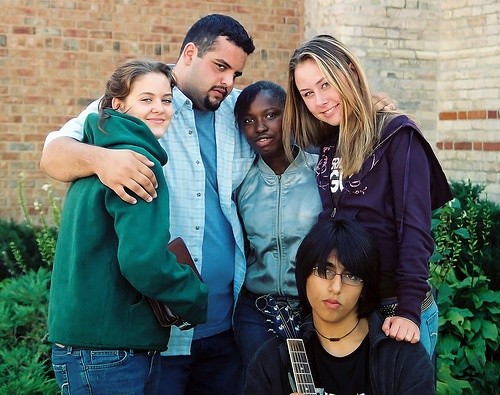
46 61 208 395
282 35 454 362
40 14 397 395
232 80 324 370
245 217 435 395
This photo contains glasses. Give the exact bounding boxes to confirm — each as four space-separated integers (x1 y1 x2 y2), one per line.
313 265 363 286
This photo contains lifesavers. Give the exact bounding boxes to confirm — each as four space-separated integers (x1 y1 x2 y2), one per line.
255 291 368 395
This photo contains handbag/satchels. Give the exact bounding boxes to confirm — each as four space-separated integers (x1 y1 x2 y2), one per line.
144 237 204 327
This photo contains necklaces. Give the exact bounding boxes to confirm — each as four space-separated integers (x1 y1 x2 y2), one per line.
313 318 361 341
